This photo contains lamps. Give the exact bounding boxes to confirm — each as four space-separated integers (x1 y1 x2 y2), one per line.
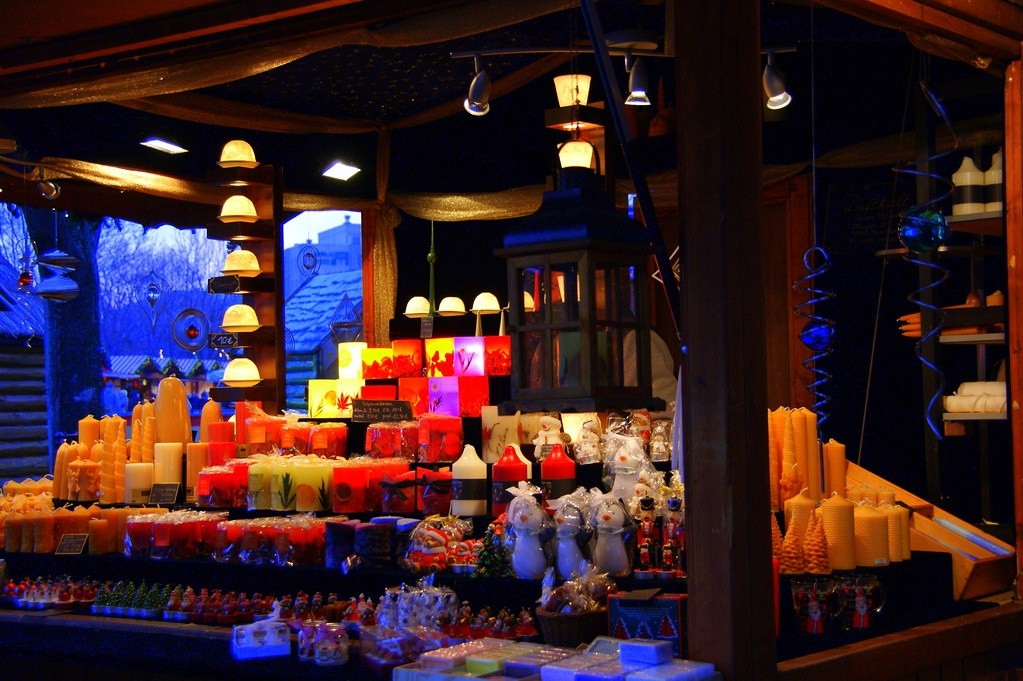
761 51 792 110
462 55 493 116
623 52 652 106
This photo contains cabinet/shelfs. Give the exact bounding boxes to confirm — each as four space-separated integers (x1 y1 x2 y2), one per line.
544 55 616 222
205 164 286 415
0 498 1023 681
918 209 1012 510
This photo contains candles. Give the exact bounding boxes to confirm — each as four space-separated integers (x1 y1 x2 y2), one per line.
0 374 603 569
767 406 911 575
896 146 1007 413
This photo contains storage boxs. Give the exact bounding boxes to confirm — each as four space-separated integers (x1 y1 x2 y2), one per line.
605 592 689 660
846 462 1018 601
